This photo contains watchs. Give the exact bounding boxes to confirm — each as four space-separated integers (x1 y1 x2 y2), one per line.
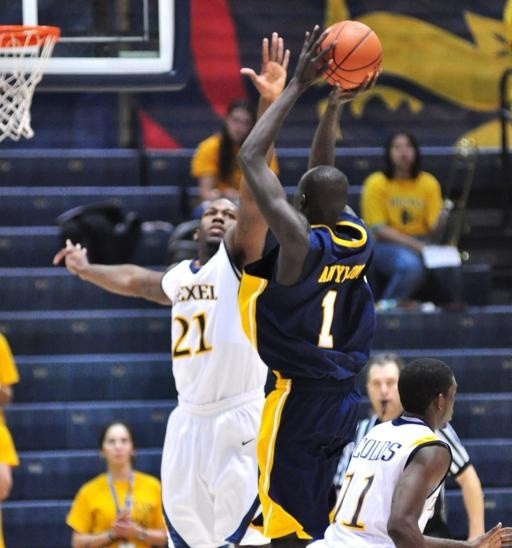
135 528 147 546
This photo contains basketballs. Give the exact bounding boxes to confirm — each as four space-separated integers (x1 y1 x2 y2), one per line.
317 20 382 90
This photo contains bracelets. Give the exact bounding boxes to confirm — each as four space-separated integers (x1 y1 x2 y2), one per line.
106 530 116 546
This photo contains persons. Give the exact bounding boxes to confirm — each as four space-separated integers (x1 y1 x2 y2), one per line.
223 22 382 548
334 352 487 542
1 329 19 409
190 95 280 218
52 31 292 548
301 357 512 548
0 412 20 548
360 129 469 316
64 421 169 547
